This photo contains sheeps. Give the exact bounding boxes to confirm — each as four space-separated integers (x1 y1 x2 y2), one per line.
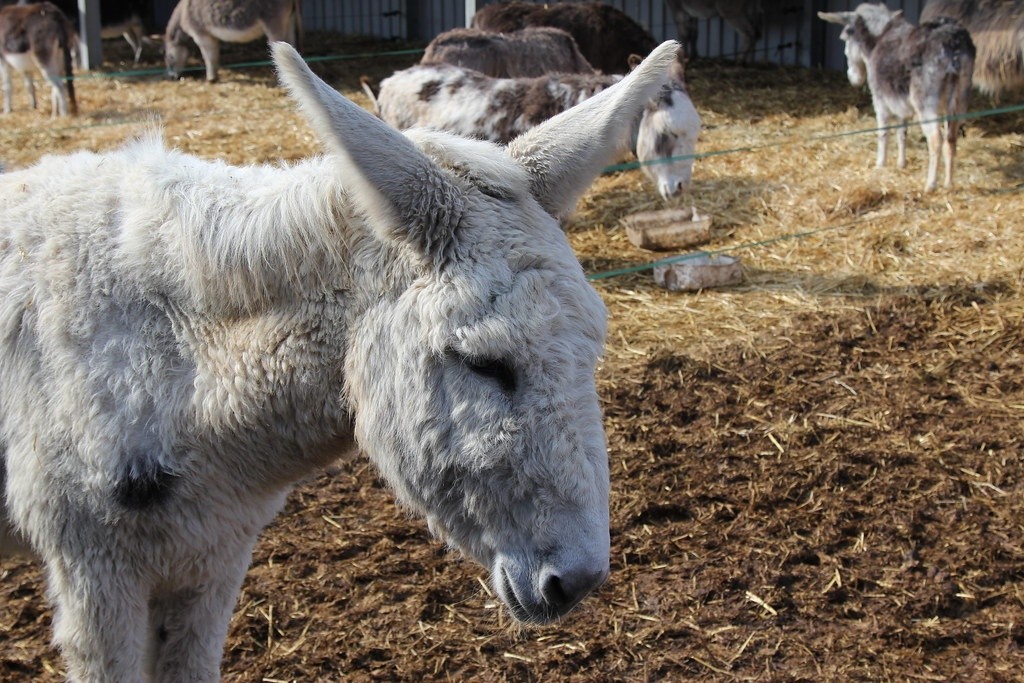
0 0 302 119
818 0 1023 194
3 41 682 683
379 1 701 203
665 0 769 71
